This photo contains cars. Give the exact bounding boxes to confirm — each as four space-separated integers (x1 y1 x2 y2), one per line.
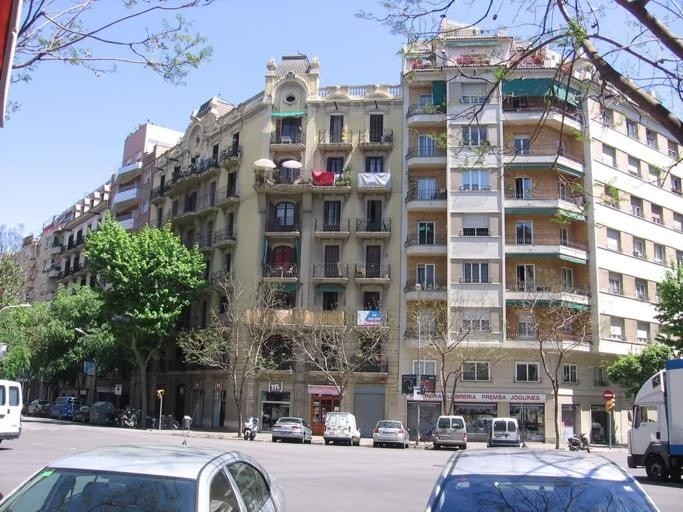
424 447 661 512
23 396 90 423
271 416 314 444
373 419 411 449
0 442 285 512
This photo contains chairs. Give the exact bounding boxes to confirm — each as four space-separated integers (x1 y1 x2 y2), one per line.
140 483 181 512
82 482 113 512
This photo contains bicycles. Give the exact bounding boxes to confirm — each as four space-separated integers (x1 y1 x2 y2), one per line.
115 406 178 429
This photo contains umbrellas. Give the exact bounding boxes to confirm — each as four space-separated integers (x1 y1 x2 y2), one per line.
254 159 276 183
282 160 302 184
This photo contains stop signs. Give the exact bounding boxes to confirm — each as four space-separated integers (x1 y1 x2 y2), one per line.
603 390 613 401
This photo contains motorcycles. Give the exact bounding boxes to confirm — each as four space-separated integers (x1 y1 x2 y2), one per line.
568 433 589 452
241 415 259 440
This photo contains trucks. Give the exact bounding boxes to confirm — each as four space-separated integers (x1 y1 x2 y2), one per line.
627 359 682 481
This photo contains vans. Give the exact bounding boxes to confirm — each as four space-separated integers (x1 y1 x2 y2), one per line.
324 411 361 446
432 414 520 449
0 379 24 443
